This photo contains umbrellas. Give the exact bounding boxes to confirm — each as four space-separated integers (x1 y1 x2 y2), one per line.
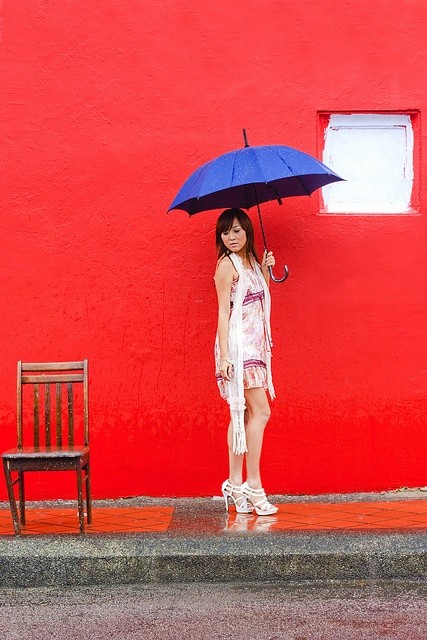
166 128 348 283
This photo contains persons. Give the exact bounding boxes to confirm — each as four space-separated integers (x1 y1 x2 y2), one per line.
213 208 280 516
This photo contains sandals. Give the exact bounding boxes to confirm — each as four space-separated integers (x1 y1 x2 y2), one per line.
241 482 278 516
221 479 254 514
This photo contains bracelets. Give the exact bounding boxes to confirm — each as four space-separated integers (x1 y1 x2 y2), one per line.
220 358 231 363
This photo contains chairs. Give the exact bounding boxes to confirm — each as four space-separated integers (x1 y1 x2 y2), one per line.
1 359 91 539
